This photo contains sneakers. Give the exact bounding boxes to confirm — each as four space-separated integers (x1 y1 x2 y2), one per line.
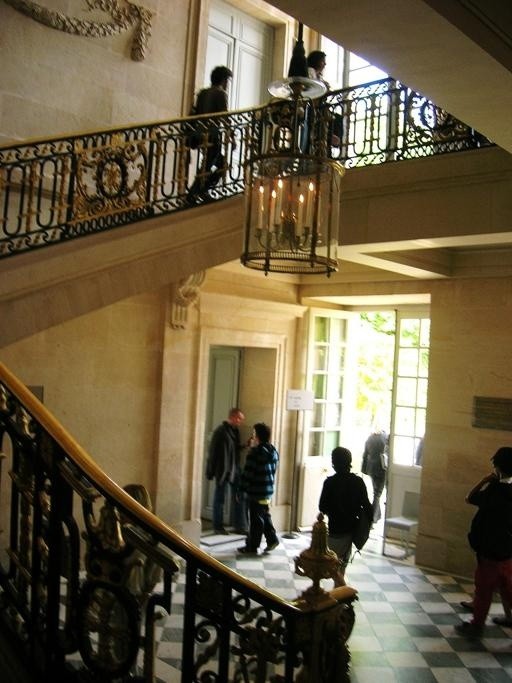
214 527 250 535
453 600 511 637
237 541 279 554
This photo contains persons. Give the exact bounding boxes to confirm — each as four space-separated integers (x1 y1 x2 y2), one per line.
237 422 280 554
181 65 238 205
82 482 163 683
204 407 253 535
319 445 374 589
304 47 343 149
361 421 391 524
453 447 511 636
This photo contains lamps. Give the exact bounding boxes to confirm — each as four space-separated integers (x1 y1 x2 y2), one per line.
239 20 345 279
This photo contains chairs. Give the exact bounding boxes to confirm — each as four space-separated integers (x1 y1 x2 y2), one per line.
382 491 421 561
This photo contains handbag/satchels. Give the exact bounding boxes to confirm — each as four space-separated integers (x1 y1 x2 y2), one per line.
352 505 372 551
